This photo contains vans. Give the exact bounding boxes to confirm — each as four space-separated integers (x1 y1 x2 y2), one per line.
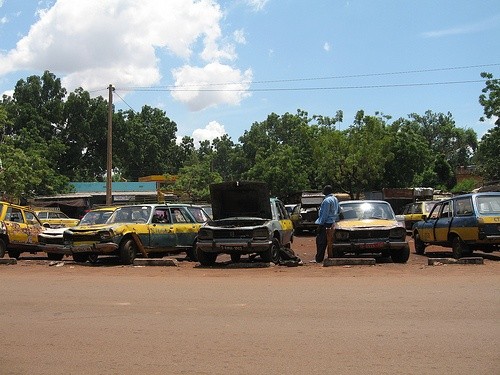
63 202 213 266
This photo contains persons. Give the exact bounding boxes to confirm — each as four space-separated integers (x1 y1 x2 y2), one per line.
309 185 341 267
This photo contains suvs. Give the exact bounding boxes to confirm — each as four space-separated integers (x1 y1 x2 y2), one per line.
0 201 43 259
194 180 295 267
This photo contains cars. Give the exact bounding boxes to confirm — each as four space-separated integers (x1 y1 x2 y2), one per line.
26 208 80 229
290 193 326 237
402 201 441 230
330 199 410 263
411 191 500 259
